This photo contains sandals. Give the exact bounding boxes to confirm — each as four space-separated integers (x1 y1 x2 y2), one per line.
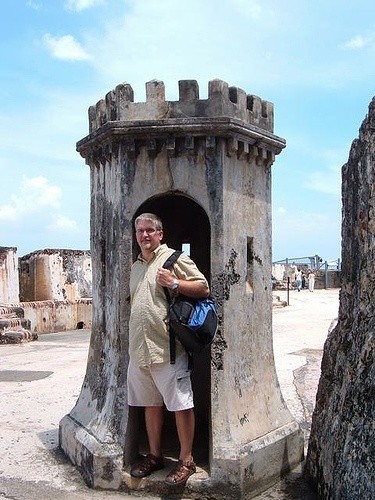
162 456 197 486
130 453 165 477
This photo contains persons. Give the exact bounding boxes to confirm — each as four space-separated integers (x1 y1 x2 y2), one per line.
302 272 306 288
126 212 211 486
308 271 315 292
296 269 302 292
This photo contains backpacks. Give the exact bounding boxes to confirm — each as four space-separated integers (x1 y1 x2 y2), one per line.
162 250 218 366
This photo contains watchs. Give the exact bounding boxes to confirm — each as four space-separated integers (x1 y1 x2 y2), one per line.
171 278 179 294
